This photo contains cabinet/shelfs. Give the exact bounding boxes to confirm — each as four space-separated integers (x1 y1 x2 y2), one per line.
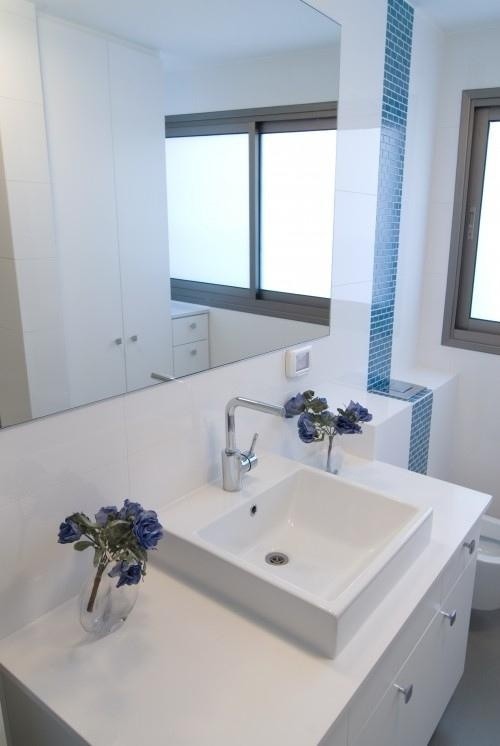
171 299 213 380
322 512 480 746
0 1 176 423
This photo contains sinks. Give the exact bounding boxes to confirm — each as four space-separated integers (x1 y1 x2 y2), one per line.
147 453 435 659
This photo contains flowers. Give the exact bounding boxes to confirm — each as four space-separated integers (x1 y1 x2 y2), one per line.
59 499 164 591
283 394 373 447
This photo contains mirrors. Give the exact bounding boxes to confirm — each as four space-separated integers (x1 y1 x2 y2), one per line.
0 0 343 432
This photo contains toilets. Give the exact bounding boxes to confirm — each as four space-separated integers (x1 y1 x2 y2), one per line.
471 514 500 611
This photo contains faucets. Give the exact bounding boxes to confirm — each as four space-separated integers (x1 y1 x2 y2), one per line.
221 397 286 492
148 371 174 383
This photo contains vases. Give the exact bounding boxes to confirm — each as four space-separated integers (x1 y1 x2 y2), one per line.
71 563 143 630
301 446 342 474
